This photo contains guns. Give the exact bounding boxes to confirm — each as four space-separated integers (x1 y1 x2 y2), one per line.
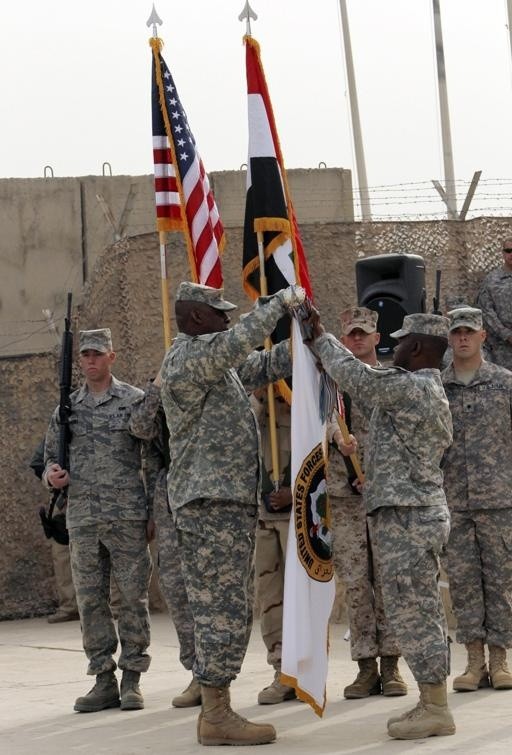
432 269 443 315
29 293 73 545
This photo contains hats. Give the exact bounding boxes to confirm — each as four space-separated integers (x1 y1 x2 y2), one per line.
176 281 237 310
339 307 378 336
390 313 450 338
78 328 113 353
446 308 482 331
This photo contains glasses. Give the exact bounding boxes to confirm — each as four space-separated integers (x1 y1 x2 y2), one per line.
503 248 512 253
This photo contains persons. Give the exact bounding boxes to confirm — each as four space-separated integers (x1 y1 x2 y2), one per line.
246 382 293 703
440 307 512 690
476 238 512 372
44 328 161 711
126 366 202 708
162 281 321 746
325 307 408 697
300 302 455 739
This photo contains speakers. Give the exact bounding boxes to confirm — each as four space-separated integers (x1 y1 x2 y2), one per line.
355 253 428 356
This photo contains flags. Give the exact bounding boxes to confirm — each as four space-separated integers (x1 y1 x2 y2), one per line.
149 37 226 289
280 284 336 720
241 35 345 422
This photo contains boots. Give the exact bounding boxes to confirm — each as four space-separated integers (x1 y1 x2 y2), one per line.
387 682 425 728
74 670 120 712
488 646 511 690
380 656 407 695
200 682 276 746
258 671 297 703
388 684 456 739
172 676 203 707
120 670 144 710
454 638 489 690
197 705 203 743
344 658 381 698
